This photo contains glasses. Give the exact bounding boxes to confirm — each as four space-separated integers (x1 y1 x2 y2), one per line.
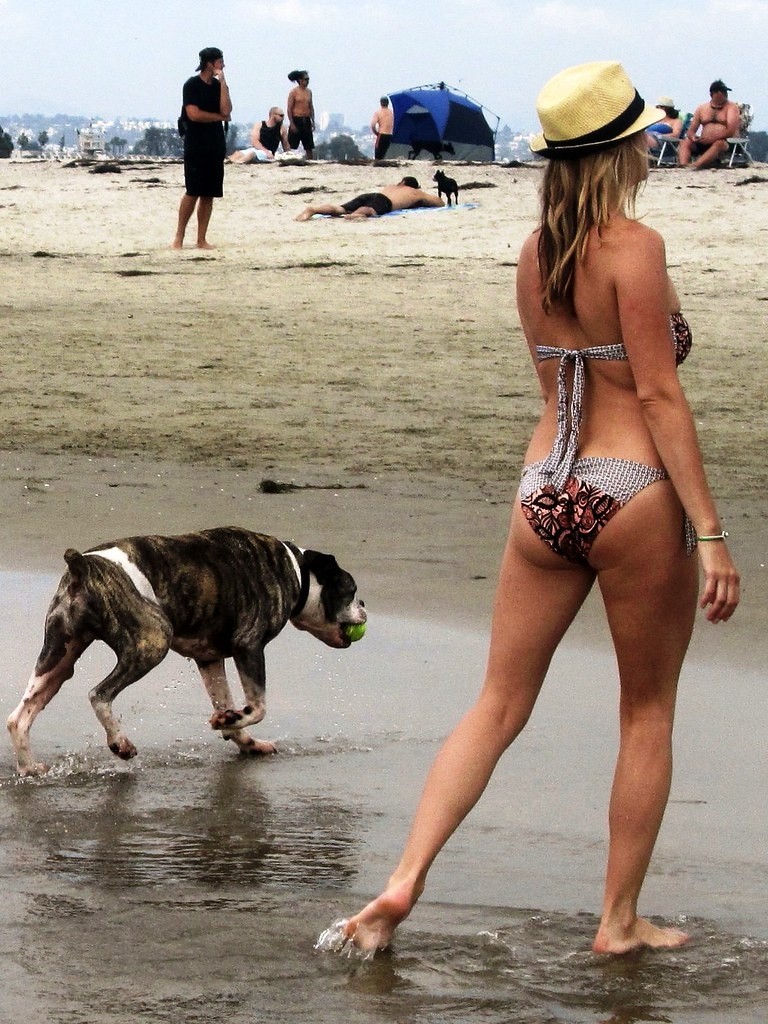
304 77 309 80
275 114 284 117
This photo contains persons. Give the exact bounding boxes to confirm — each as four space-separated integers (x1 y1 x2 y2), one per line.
677 79 740 169
294 176 445 220
343 59 749 955
645 98 682 148
171 47 233 249
371 96 394 160
286 70 315 162
226 107 290 163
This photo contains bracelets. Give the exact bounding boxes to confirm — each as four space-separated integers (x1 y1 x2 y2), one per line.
695 531 728 542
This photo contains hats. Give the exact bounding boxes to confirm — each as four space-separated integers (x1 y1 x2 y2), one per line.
709 80 732 93
530 62 666 161
195 47 223 72
655 96 675 109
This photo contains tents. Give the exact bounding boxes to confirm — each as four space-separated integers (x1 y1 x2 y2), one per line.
383 80 502 162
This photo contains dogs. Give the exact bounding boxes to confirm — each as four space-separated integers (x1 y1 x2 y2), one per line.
8 526 369 778
431 170 459 210
408 132 456 160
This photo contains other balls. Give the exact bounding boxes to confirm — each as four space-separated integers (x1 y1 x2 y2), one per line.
344 622 366 642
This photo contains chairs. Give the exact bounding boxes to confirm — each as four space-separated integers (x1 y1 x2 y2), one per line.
685 103 753 168
645 113 694 167
